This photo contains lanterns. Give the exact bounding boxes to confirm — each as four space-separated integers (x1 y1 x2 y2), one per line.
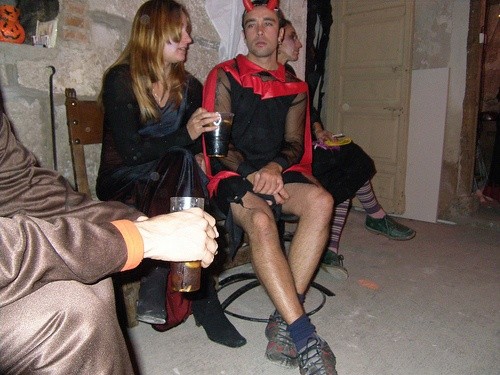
0 20 25 44
0 4 20 21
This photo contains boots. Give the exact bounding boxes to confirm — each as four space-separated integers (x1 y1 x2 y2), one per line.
134 260 170 324
189 276 246 348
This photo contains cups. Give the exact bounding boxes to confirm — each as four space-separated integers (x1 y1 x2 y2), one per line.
205 112 234 158
168 196 205 292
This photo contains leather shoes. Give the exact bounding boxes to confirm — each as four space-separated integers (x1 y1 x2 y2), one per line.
319 249 349 281
364 212 417 239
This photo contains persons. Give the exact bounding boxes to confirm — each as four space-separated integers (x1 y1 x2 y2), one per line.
202 0 338 375
0 110 219 375
95 0 248 348
277 19 416 281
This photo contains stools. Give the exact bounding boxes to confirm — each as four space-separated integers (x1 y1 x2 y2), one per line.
213 193 335 323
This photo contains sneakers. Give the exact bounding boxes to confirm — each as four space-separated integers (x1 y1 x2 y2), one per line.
265 314 297 369
296 333 337 375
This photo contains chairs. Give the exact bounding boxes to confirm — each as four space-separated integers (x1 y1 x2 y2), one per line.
64 88 143 330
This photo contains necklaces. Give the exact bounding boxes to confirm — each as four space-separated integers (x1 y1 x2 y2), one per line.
153 88 161 106
